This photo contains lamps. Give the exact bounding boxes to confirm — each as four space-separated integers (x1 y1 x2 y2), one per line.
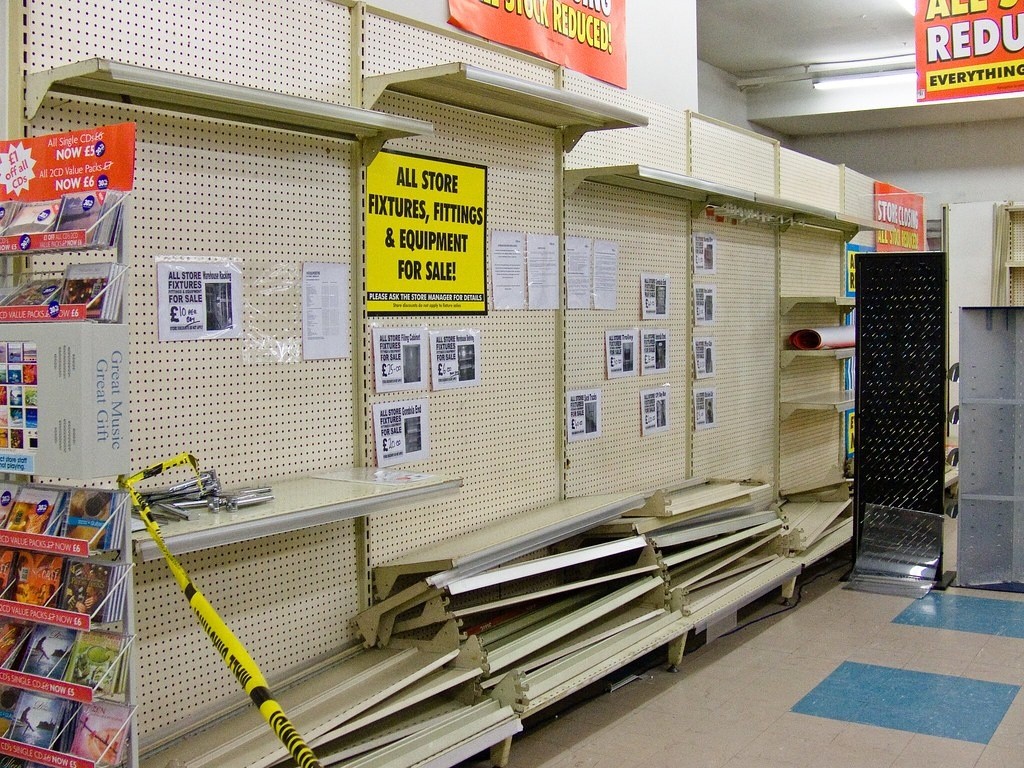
812 68 917 90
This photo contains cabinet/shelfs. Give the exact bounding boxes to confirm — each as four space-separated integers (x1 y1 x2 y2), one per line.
0 0 1024 768
